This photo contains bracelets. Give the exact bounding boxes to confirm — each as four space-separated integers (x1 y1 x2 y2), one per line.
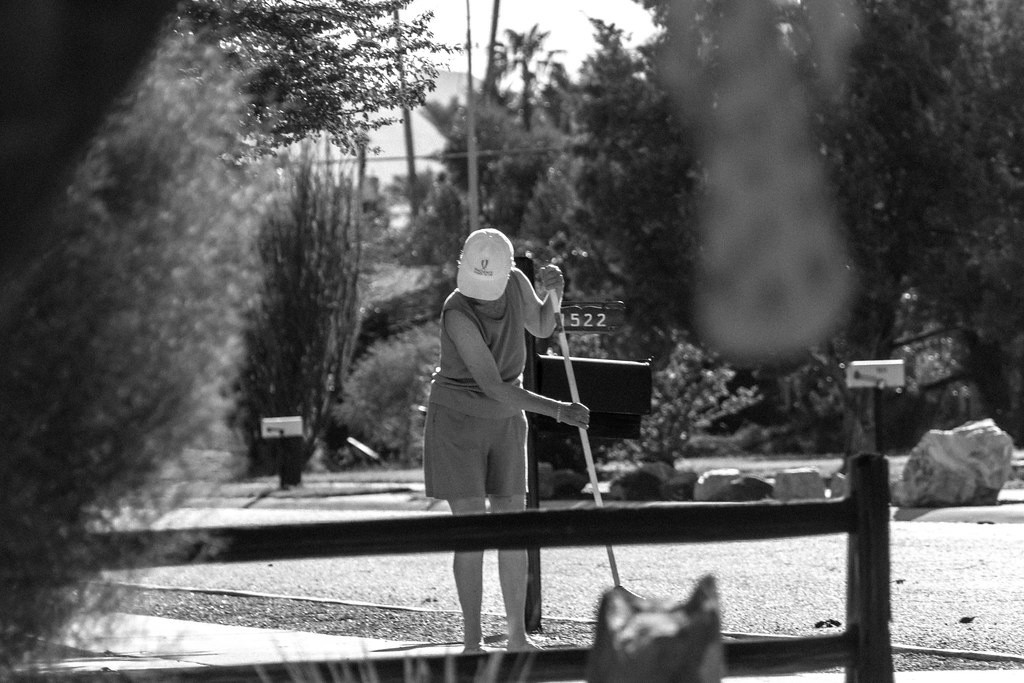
556 400 561 423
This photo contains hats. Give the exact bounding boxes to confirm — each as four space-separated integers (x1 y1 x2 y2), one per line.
456 228 515 302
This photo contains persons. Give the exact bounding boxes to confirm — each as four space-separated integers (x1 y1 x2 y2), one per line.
424 228 590 656
